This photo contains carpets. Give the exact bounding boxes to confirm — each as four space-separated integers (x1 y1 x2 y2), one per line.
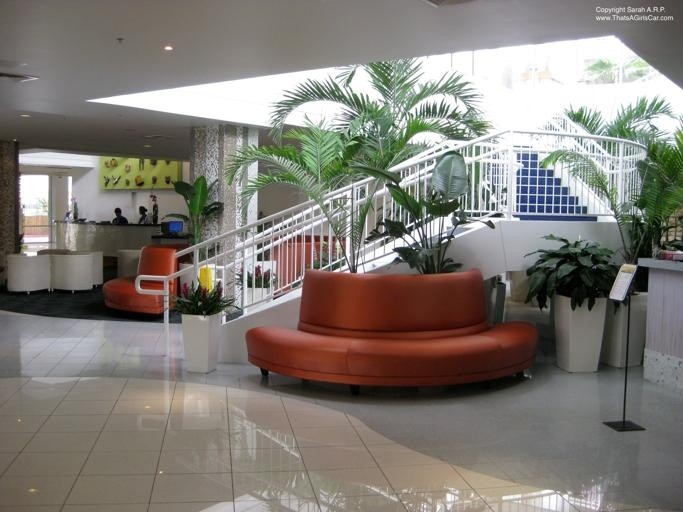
0 286 183 325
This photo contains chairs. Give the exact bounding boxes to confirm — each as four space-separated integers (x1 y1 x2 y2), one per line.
101 245 178 317
4 249 104 295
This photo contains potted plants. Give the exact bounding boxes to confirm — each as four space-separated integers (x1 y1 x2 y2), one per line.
523 134 682 374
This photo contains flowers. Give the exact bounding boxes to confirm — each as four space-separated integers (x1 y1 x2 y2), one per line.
175 280 234 316
238 268 276 288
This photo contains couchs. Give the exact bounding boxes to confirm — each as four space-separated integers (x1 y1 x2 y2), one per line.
241 266 537 396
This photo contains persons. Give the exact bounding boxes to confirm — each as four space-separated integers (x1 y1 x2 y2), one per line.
138 206 151 224
112 208 128 224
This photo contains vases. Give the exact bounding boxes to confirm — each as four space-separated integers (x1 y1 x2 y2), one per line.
181 311 222 375
246 286 271 306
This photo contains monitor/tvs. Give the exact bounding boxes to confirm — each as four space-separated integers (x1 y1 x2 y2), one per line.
168 220 183 233
